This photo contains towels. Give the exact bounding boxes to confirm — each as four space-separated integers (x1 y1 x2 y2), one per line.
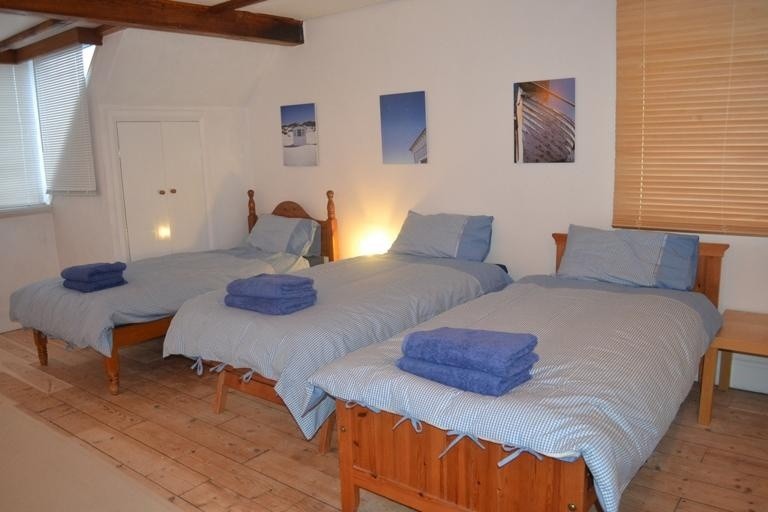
397 326 537 398
224 273 318 316
60 261 129 291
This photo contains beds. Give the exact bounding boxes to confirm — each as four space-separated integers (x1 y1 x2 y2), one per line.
162 210 513 454
10 190 340 395
307 232 728 512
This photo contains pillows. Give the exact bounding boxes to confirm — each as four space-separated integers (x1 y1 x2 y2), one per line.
387 210 494 261
557 224 699 291
248 213 319 257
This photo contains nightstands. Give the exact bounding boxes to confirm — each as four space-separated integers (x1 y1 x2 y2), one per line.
697 308 768 425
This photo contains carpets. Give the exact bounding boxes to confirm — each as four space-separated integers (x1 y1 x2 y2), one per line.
0 317 768 512
